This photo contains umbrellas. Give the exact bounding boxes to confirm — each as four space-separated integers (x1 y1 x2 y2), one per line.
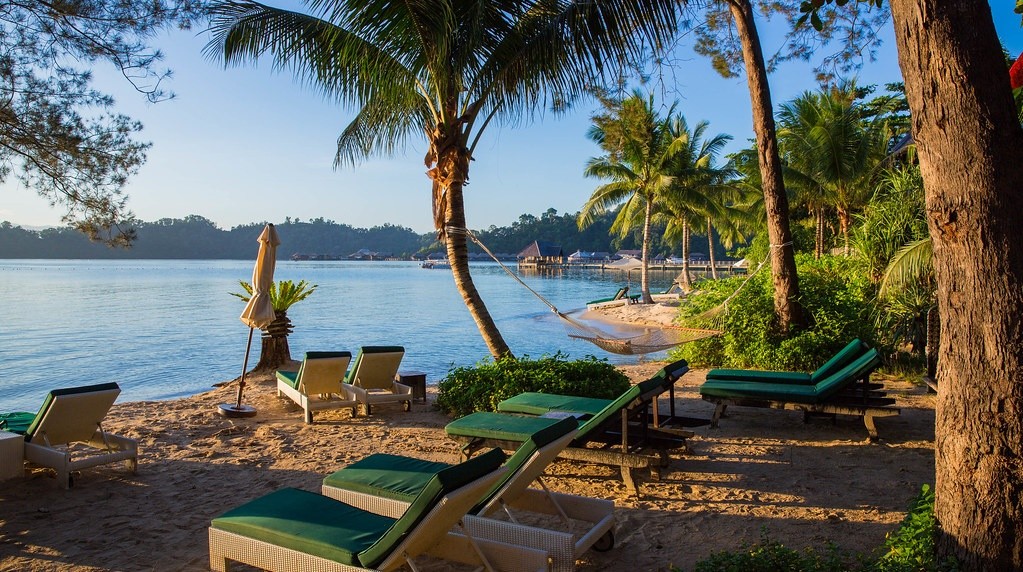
733 259 750 269
604 256 642 303
217 222 278 418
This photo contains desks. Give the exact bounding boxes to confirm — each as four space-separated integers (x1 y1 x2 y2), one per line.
0 429 24 483
397 372 427 403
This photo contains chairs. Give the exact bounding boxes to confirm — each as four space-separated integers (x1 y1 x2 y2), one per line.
275 352 357 423
344 345 413 416
208 446 554 572
444 376 684 493
700 347 901 441
0 382 138 490
498 359 710 453
586 287 642 305
706 338 887 397
321 375 616 572
